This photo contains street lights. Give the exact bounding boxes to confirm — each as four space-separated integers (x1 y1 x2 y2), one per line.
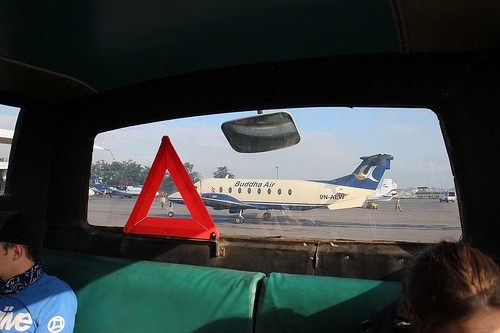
275 166 279 180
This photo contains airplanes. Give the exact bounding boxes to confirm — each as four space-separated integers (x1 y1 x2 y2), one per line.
166 153 398 224
90 173 160 199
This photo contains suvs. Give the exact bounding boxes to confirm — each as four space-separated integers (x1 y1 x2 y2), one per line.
439 191 456 203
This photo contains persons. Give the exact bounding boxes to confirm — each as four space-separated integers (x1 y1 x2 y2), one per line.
352 239 500 333
0 212 78 333
160 195 166 209
395 197 402 212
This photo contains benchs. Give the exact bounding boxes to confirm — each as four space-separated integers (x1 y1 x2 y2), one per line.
31 249 404 333
